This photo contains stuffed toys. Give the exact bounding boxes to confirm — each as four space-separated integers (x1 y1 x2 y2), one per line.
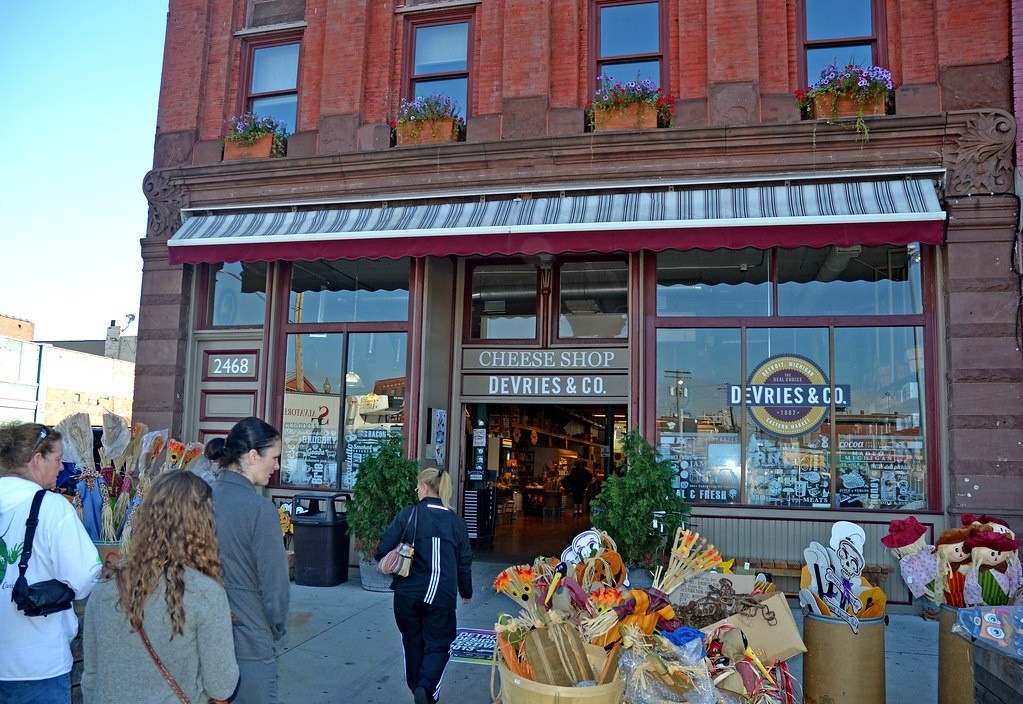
929 509 1023 613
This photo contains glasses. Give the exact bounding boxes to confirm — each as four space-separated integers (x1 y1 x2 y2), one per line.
32 423 50 453
415 484 423 494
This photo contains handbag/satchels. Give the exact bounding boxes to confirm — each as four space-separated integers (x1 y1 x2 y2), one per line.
377 505 419 576
11 490 76 618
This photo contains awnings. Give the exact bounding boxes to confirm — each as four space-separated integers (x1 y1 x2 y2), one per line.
165 163 948 265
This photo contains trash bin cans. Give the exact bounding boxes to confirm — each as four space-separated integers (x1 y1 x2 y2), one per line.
289 493 351 587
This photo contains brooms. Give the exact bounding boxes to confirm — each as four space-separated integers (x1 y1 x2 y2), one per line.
97 412 204 543
52 412 115 542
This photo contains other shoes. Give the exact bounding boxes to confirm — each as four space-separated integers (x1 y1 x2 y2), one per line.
579 512 583 518
574 513 578 518
413 686 429 704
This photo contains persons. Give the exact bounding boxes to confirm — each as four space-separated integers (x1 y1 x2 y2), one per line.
79 469 241 704
205 417 290 704
374 467 473 704
0 422 102 704
567 457 593 518
